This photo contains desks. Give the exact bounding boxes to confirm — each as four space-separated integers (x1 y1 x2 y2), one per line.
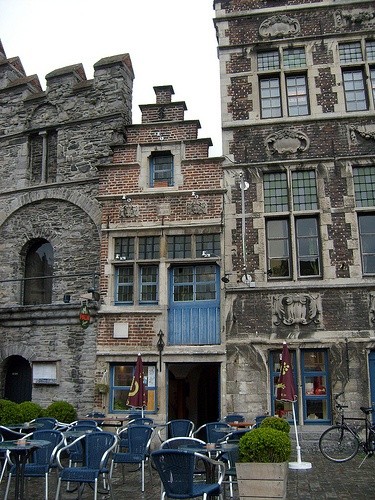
228 421 256 428
61 430 110 495
211 428 251 444
179 444 240 500
80 414 130 490
0 439 51 500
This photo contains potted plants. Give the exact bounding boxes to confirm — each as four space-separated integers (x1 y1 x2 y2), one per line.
236 428 292 500
97 384 110 394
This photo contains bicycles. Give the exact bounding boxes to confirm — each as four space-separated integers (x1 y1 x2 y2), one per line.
319 392 375 469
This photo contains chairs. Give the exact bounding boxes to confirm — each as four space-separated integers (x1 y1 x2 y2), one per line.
0 412 268 500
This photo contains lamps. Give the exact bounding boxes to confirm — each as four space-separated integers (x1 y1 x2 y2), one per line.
157 329 165 371
